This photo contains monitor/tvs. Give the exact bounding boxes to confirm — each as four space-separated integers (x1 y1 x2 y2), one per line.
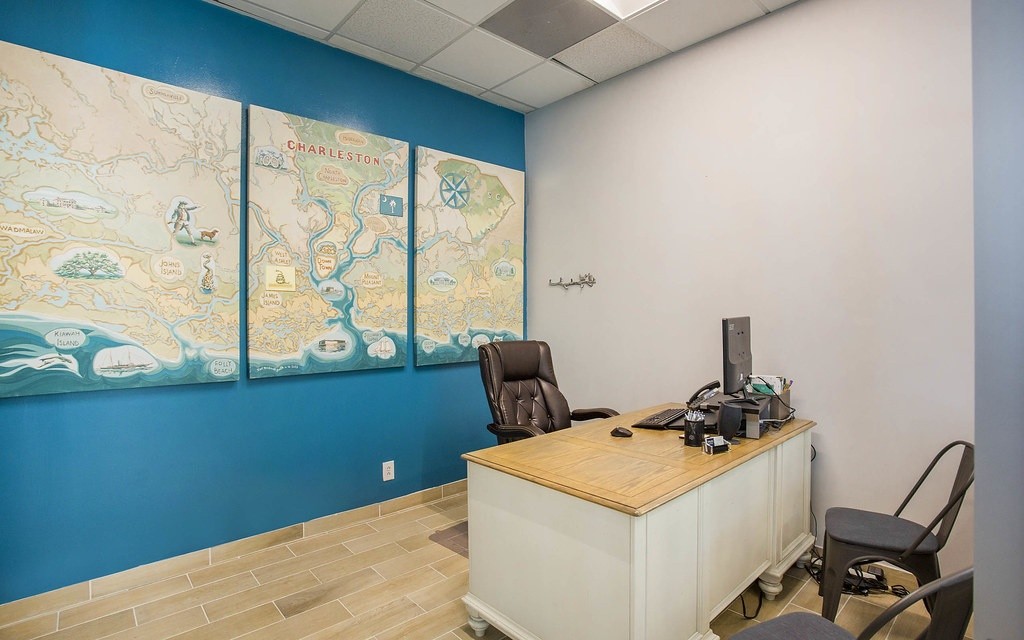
722 315 753 396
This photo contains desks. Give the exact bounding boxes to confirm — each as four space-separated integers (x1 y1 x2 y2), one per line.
459 401 817 640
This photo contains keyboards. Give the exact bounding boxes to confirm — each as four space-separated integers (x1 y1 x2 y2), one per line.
632 408 690 428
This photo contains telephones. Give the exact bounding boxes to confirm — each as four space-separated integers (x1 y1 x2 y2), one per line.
686 380 720 411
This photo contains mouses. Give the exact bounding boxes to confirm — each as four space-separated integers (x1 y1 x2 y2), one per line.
610 426 633 437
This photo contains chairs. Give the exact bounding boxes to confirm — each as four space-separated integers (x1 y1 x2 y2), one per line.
817 440 975 623
477 340 620 445
729 563 974 640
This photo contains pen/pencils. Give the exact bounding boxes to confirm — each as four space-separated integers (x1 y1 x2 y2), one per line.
684 410 705 435
787 379 793 390
783 378 786 390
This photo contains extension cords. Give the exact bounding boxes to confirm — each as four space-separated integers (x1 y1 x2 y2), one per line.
812 557 879 580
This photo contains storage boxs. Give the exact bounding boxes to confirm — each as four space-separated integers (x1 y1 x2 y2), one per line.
770 390 791 421
